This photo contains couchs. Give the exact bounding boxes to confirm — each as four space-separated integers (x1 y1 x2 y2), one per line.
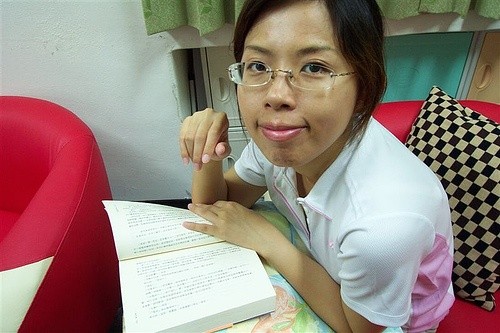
372 99 500 332
0 95 120 333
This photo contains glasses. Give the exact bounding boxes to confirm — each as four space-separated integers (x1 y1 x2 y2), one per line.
224 62 356 90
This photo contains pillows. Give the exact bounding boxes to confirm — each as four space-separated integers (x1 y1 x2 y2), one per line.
403 85 500 312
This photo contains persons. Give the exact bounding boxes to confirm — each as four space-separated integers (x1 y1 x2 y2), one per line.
179 1 455 332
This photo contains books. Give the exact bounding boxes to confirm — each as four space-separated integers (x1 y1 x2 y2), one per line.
101 200 276 332
